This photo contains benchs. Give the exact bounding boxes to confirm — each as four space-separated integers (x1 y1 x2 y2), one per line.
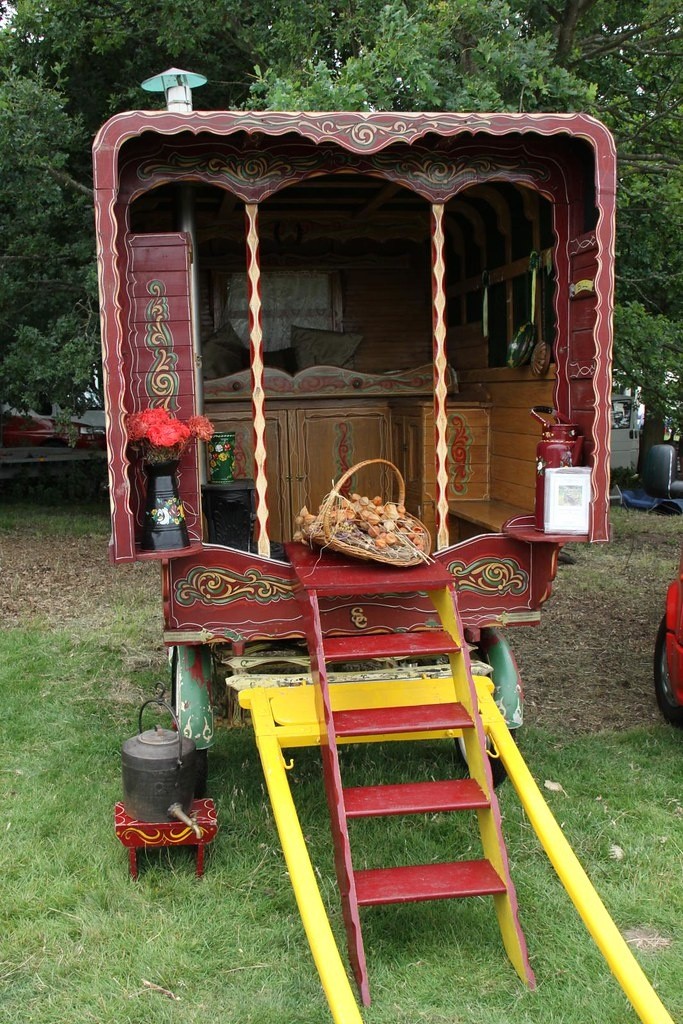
439 368 558 536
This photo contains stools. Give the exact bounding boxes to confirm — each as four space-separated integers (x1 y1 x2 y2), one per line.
201 478 255 555
114 799 220 879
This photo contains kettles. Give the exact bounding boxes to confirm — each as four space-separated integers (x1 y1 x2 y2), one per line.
121 682 202 840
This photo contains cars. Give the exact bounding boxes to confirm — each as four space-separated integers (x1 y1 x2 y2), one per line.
0 380 106 428
1 413 108 449
653 547 683 735
607 395 641 473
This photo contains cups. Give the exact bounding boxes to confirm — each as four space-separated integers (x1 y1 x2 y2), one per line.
205 431 237 484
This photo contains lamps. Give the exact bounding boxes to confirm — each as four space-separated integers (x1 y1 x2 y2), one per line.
142 67 207 114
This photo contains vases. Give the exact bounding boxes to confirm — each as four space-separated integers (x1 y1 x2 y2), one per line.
141 460 192 553
209 431 235 483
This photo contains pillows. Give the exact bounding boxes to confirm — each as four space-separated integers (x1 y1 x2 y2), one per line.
291 324 365 378
201 323 249 380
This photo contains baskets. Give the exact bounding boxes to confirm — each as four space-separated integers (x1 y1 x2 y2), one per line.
302 460 432 566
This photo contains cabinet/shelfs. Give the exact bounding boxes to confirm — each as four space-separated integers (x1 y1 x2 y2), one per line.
206 398 489 553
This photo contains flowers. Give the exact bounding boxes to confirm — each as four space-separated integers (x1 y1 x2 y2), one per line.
127 406 213 462
